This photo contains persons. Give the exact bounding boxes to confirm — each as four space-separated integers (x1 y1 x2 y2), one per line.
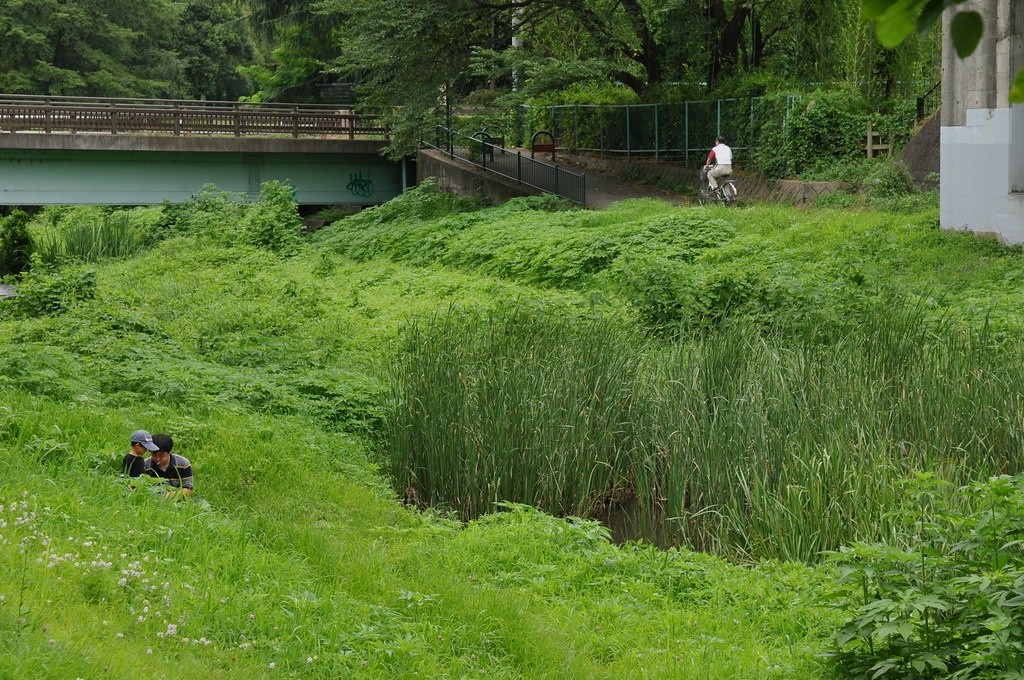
705 134 733 206
121 428 159 477
143 433 194 499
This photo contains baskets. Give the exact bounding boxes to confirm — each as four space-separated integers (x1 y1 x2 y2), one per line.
699 168 712 182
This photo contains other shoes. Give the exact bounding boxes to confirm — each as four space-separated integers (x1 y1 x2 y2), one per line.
709 186 720 194
720 195 731 200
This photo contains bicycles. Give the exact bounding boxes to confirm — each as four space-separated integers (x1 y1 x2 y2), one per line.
697 165 737 207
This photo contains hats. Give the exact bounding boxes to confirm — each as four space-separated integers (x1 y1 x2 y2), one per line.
131 430 160 451
